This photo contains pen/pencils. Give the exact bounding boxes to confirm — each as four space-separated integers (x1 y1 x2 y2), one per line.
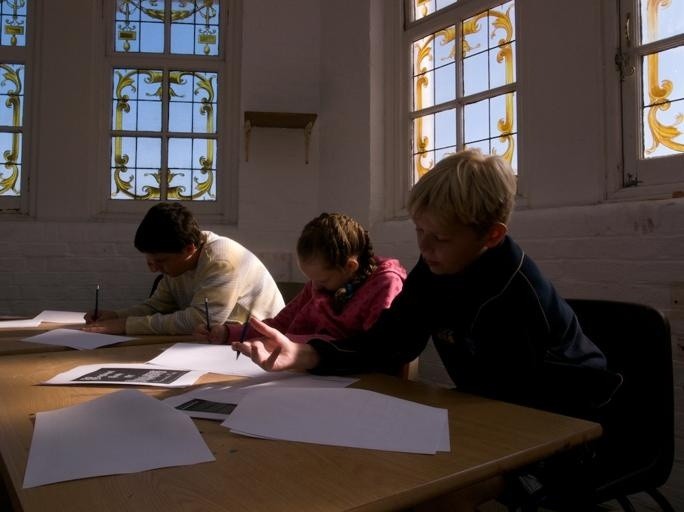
205 298 212 344
237 298 255 359
94 285 100 322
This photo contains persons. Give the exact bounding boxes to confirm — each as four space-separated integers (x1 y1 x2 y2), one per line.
79 201 289 338
230 141 631 512
194 210 409 352
142 254 164 299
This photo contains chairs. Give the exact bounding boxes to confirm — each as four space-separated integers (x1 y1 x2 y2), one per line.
564 297 677 512
277 280 305 304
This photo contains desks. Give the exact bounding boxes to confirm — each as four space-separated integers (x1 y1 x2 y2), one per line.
0 313 604 512
